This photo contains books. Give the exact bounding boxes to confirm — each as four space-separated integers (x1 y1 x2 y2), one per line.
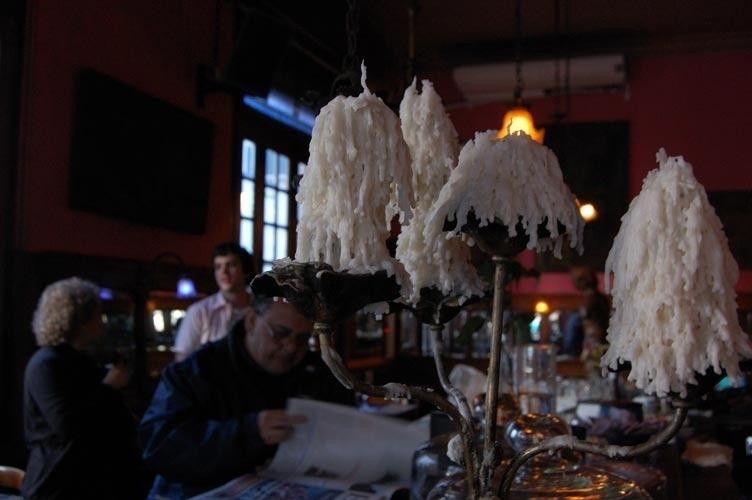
180 393 422 500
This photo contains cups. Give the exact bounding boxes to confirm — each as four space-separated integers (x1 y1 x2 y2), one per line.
512 344 557 415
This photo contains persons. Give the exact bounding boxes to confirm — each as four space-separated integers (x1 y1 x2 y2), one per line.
585 362 733 497
167 239 257 363
140 286 360 499
564 291 613 363
21 275 137 499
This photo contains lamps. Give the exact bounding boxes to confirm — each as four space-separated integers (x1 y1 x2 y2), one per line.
497 0 544 144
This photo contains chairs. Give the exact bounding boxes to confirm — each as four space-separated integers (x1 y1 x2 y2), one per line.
0 467 26 500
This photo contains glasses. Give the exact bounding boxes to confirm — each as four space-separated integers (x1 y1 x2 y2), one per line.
254 309 309 347
